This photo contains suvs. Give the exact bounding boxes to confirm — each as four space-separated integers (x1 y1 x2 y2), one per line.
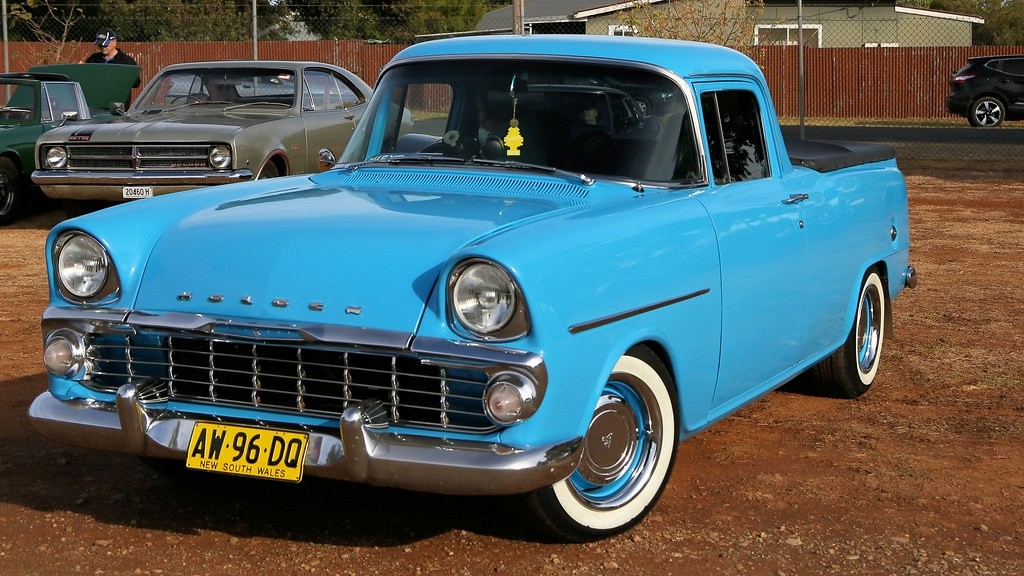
943 54 1024 128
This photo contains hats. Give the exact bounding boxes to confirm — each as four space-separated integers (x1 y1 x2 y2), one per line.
93 29 116 46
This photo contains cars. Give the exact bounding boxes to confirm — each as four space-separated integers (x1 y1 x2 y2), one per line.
30 60 414 214
0 63 143 227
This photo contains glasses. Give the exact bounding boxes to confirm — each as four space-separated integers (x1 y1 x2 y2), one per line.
211 84 234 91
580 105 596 111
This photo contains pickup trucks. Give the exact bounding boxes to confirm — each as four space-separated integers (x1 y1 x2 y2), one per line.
27 34 919 543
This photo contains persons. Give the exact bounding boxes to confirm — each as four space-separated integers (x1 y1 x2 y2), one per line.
579 102 599 125
78 27 141 116
